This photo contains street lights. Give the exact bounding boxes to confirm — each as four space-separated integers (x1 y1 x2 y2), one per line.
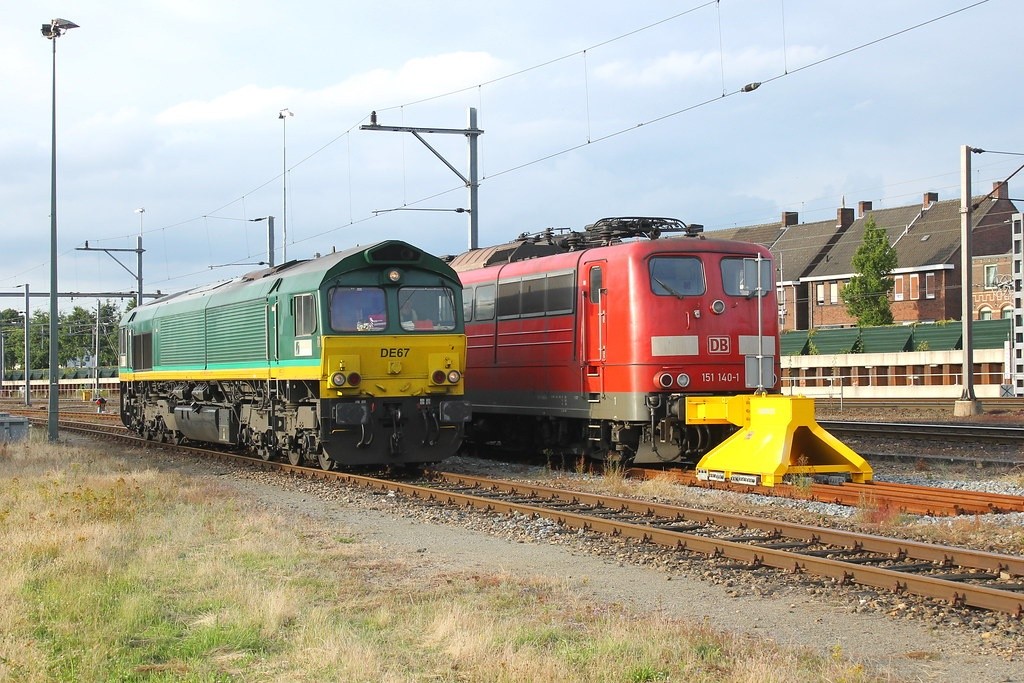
41 17 86 447
15 283 31 409
278 107 294 261
253 215 277 268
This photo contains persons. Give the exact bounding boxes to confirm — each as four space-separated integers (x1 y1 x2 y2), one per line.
400 297 418 321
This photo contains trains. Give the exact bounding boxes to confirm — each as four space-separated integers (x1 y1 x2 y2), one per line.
436 216 782 465
117 240 471 473
